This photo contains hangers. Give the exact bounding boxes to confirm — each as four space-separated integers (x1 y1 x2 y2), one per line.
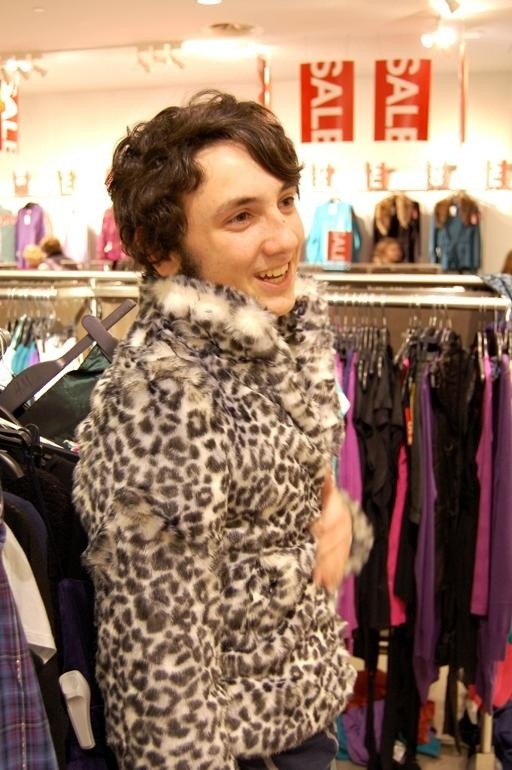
317 289 510 406
1 280 140 485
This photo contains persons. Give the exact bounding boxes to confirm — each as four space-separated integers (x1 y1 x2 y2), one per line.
69 88 376 769
